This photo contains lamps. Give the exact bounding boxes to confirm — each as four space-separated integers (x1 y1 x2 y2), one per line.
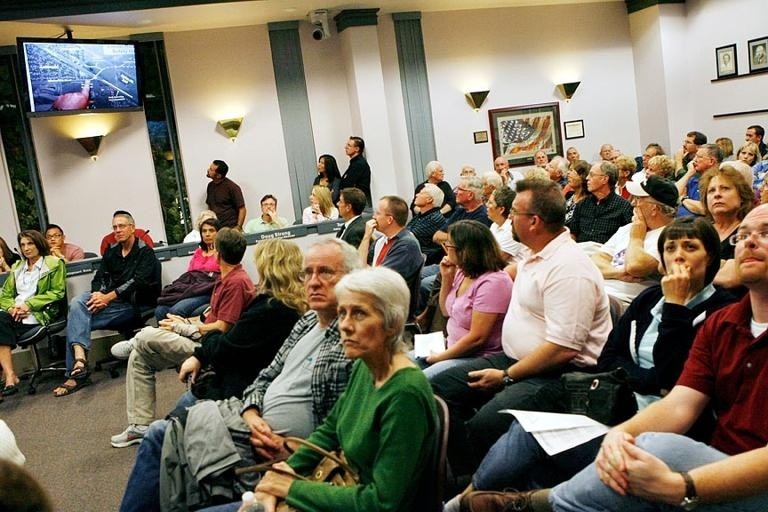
75 135 104 162
556 81 580 104
217 117 243 142
465 92 489 113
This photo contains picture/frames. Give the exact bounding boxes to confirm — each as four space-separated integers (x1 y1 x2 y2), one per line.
715 43 739 79
747 35 768 75
488 102 564 168
474 131 489 145
564 119 585 141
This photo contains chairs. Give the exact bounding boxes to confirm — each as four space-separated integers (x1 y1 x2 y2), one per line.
16 287 68 395
426 391 451 512
608 296 623 325
95 258 162 379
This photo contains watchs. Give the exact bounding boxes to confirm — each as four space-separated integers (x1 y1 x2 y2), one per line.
677 470 700 511
501 368 510 387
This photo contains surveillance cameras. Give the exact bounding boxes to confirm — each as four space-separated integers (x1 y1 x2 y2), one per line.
311 28 325 42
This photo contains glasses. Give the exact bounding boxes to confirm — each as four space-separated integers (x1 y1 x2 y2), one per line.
44 233 62 240
297 269 335 282
729 229 768 246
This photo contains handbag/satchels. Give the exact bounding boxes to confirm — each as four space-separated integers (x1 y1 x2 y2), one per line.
560 366 640 427
275 437 360 512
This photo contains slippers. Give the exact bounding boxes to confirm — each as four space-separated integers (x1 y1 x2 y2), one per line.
4 383 16 395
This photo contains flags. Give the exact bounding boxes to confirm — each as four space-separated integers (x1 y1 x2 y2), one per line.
500 115 554 156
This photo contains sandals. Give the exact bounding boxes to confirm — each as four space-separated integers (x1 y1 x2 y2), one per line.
54 377 92 397
70 358 89 380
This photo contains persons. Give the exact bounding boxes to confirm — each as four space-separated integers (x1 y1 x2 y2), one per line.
558 166 755 327
429 175 614 499
1 417 57 512
107 160 309 449
520 143 637 253
719 53 733 71
114 235 362 512
0 210 161 397
753 45 767 65
436 214 740 512
401 143 526 377
187 263 441 512
636 124 768 204
300 135 424 329
458 203 768 511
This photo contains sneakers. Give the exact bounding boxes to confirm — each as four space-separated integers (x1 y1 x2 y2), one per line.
110 339 132 358
110 424 151 448
460 488 537 512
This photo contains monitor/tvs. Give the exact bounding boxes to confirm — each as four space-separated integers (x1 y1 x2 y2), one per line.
16 37 143 118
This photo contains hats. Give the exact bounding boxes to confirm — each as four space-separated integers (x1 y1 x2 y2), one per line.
196 210 218 226
625 176 680 209
